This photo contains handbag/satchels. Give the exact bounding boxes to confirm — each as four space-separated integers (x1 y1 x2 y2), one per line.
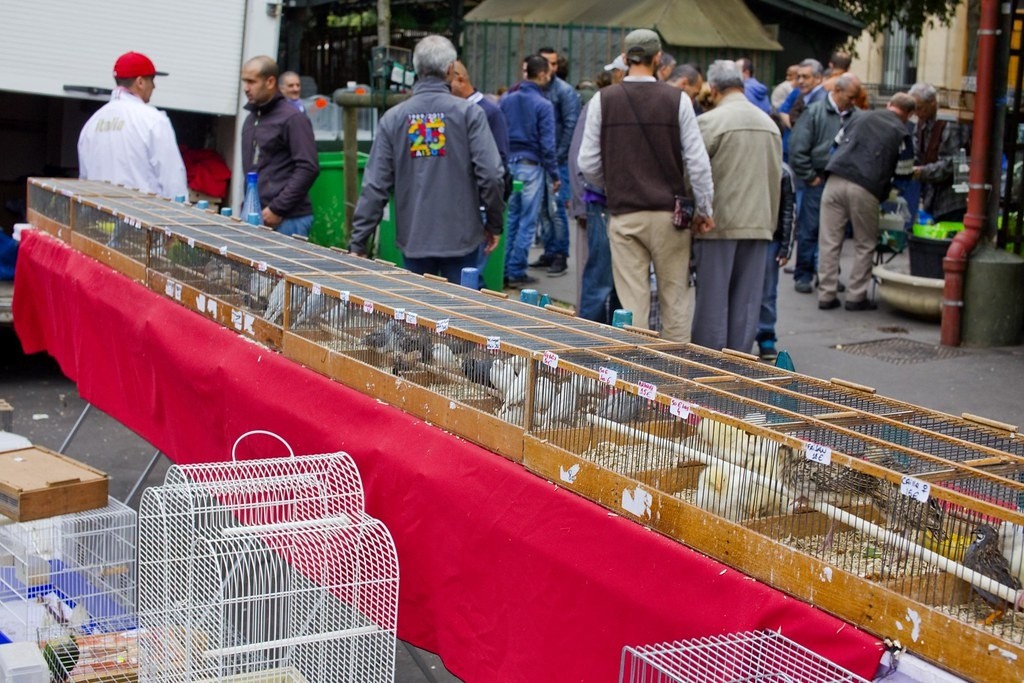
673 196 695 230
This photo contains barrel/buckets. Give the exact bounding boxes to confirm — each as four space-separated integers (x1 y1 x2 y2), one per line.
904 224 952 278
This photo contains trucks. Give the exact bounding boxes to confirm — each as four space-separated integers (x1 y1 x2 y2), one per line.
1 1 281 320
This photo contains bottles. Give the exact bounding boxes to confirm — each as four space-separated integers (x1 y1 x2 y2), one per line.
176 170 265 227
332 81 380 142
303 95 339 141
955 146 970 185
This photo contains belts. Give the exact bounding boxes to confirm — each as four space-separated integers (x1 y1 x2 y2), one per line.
517 159 539 166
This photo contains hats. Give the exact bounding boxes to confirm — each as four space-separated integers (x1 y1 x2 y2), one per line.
603 54 629 71
625 29 662 57
113 51 169 78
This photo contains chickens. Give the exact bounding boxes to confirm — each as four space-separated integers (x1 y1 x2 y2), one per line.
696 413 806 521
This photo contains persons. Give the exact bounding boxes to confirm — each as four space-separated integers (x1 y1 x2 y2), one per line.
278 71 306 115
347 35 504 286
576 29 716 343
497 54 562 284
77 50 191 204
240 54 320 240
682 58 783 354
818 91 917 311
451 60 514 290
451 28 967 361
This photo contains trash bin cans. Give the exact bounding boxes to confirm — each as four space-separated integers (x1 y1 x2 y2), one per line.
378 195 406 269
307 150 369 251
480 180 524 293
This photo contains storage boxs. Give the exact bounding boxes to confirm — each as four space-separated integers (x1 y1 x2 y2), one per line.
0 444 108 520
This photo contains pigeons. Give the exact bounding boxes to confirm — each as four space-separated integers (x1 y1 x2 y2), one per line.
358 319 648 430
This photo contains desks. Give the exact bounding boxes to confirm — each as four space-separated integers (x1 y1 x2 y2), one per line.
12 219 977 682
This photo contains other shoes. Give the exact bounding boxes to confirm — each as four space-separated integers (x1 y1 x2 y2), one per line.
508 275 540 287
818 298 842 309
795 279 812 293
758 342 778 359
529 256 568 277
845 300 880 312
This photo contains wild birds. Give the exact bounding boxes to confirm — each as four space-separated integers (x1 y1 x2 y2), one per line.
45 194 347 329
995 520 1023 589
778 445 879 552
963 524 1023 624
44 633 80 683
871 458 949 544
